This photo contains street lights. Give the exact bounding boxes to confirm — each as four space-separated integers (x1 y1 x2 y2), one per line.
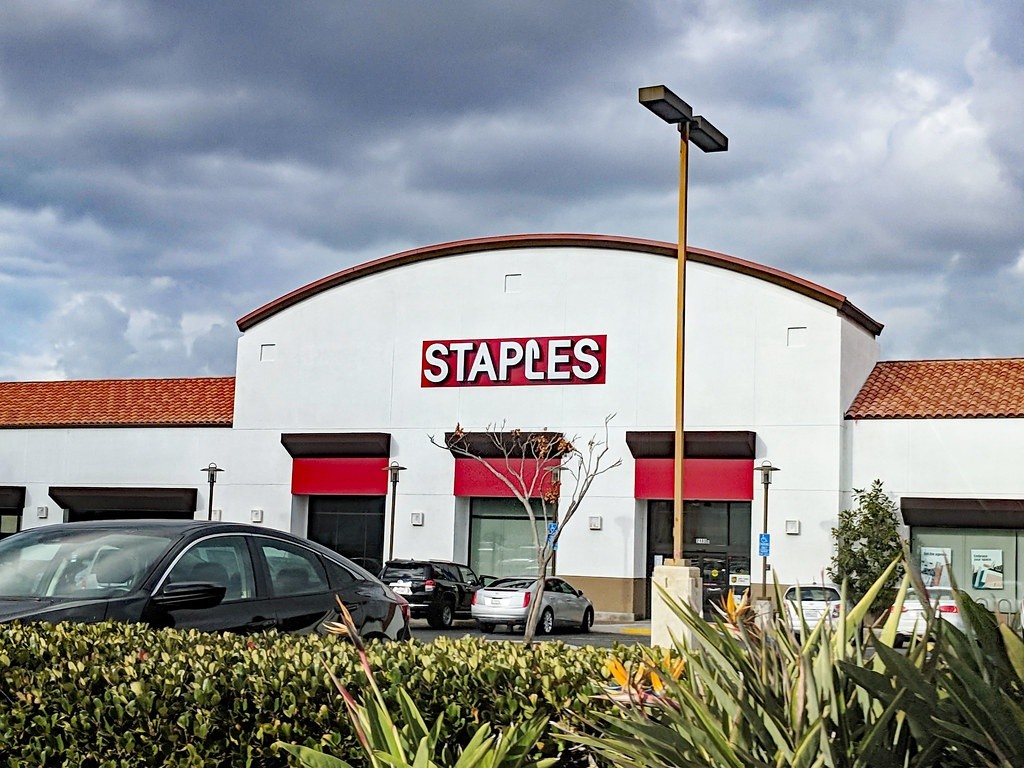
543 465 570 577
382 461 408 564
752 460 781 628
638 84 728 671
201 462 225 521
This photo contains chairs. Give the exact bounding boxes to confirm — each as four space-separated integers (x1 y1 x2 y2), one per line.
96 550 132 584
169 563 243 593
275 568 310 594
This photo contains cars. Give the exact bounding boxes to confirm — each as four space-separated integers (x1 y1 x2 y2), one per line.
0 519 415 646
779 584 864 648
470 575 594 636
889 586 977 648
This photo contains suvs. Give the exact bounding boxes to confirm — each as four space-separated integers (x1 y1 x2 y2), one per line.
377 558 485 630
